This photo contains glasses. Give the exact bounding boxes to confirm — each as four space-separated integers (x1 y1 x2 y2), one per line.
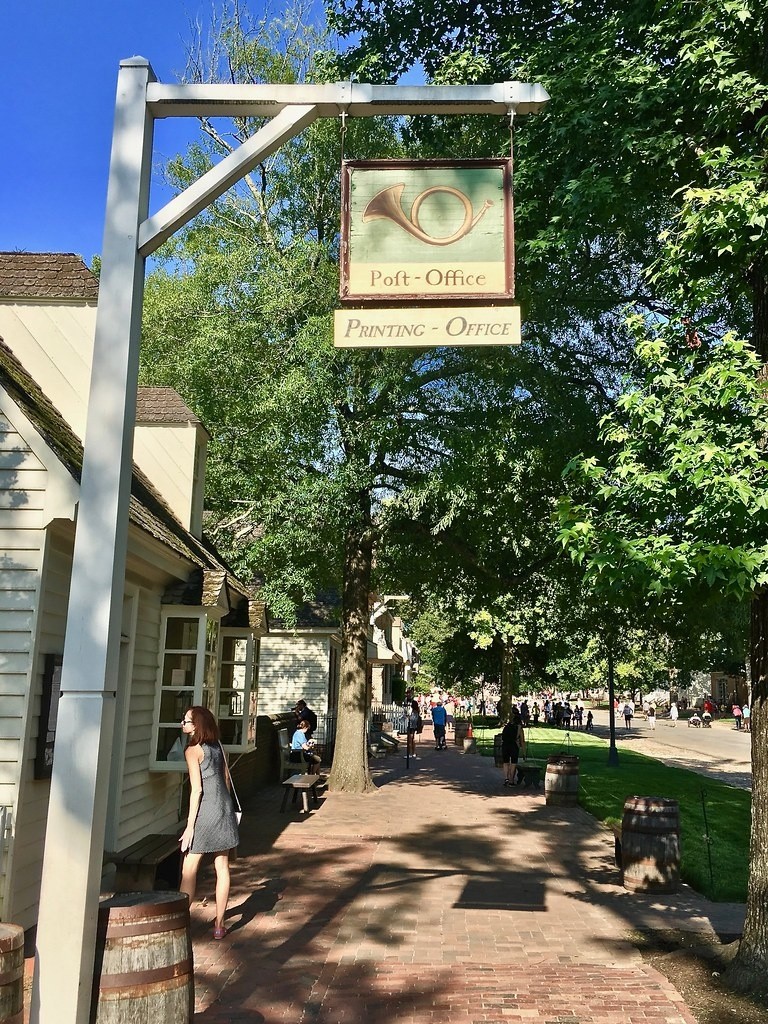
183 721 193 725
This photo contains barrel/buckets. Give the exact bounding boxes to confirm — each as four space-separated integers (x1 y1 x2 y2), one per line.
494 735 504 767
0 922 24 1024
545 762 578 807
455 723 468 746
87 891 196 1024
622 794 680 895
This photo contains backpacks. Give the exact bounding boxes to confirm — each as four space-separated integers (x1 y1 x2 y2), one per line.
502 723 519 744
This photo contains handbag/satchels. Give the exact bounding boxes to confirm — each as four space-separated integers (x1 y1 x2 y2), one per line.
417 716 422 734
234 811 243 826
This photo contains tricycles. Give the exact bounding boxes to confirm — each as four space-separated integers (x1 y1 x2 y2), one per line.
688 719 700 728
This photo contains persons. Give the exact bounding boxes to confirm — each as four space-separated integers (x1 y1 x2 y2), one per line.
404 700 420 758
180 707 240 939
502 715 526 787
743 705 750 732
660 697 688 713
643 699 657 730
513 697 541 728
703 699 724 713
541 692 584 728
690 713 701 722
703 710 711 727
486 701 497 715
417 693 473 731
733 706 741 730
614 699 635 730
670 703 679 727
431 703 447 750
586 711 593 730
295 699 313 740
291 720 327 783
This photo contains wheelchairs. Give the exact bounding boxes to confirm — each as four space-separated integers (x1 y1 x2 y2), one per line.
703 716 712 728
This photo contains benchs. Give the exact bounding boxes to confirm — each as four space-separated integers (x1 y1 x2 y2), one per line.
515 763 542 790
277 726 318 782
280 774 319 813
112 832 184 893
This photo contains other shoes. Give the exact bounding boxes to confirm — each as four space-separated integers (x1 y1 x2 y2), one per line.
214 927 227 940
503 779 509 784
509 783 516 787
404 755 412 758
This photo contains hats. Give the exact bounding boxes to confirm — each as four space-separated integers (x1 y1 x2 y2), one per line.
671 703 675 706
